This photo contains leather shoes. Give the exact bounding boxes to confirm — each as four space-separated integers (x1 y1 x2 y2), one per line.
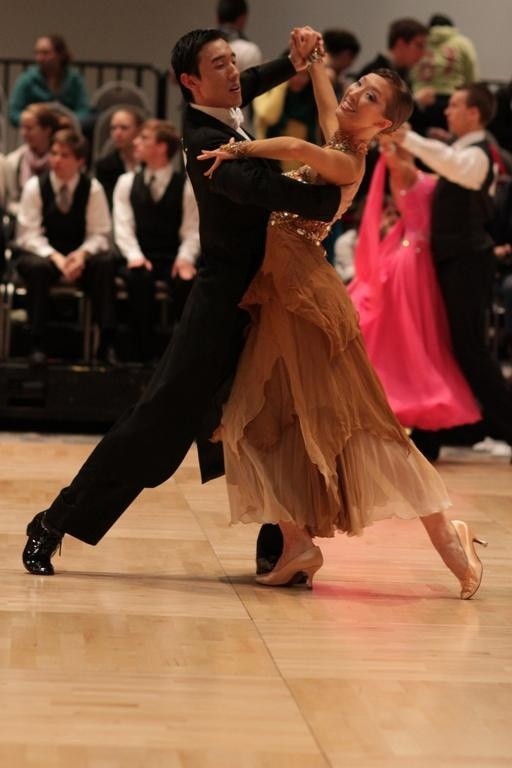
23 510 61 574
257 555 308 584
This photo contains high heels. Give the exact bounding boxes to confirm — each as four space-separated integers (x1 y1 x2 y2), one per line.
451 519 488 599
256 545 323 589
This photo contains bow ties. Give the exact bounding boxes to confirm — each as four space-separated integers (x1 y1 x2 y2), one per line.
229 107 244 129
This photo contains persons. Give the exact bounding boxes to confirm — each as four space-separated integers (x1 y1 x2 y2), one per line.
13 128 119 369
93 108 143 214
196 30 489 599
6 33 90 147
111 117 201 362
5 104 76 221
195 1 498 463
22 25 356 584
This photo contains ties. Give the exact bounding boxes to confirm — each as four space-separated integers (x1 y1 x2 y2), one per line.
147 177 154 188
60 185 69 214
